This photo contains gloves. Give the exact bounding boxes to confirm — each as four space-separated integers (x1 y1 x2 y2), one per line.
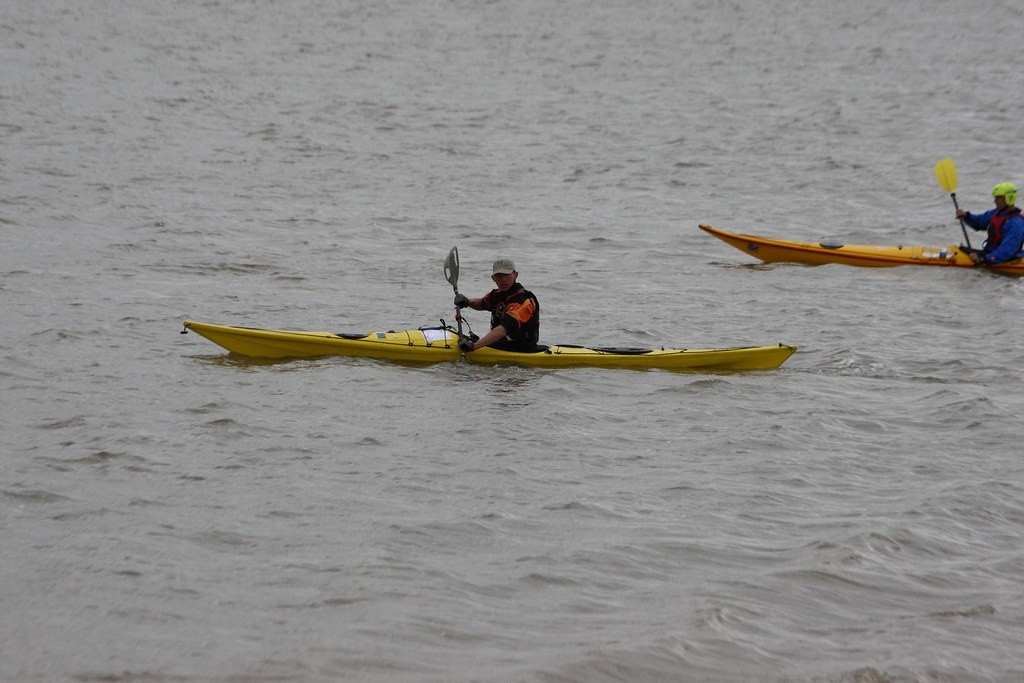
458 338 474 352
454 294 469 309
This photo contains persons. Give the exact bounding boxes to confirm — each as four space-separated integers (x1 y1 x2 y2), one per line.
454 257 546 353
955 182 1024 266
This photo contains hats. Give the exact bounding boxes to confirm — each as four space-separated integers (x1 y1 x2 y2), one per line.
491 258 516 276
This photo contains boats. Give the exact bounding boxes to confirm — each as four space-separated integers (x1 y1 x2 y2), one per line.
698 224 1024 276
178 320 798 374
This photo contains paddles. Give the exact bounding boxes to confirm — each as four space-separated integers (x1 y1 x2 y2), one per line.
442 247 468 377
934 155 979 272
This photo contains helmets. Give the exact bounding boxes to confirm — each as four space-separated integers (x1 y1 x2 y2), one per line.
992 182 1018 207
